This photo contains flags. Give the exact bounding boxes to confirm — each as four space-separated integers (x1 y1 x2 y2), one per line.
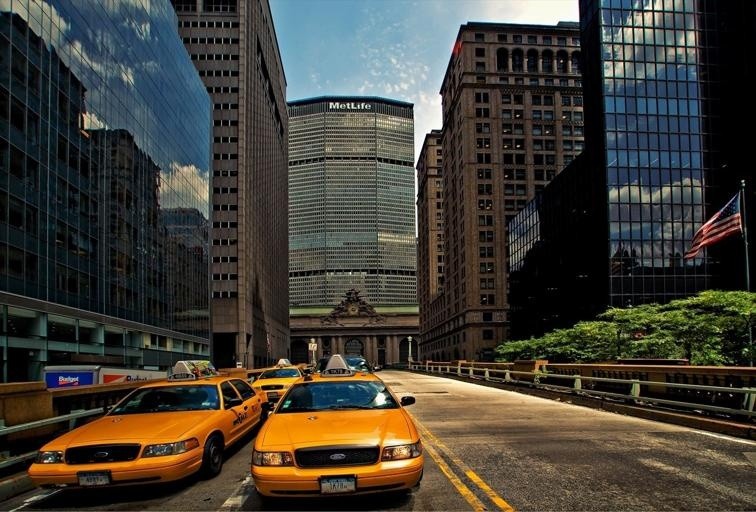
683 188 744 261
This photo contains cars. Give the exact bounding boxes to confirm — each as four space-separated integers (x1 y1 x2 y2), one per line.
251 354 424 501
26 359 269 491
